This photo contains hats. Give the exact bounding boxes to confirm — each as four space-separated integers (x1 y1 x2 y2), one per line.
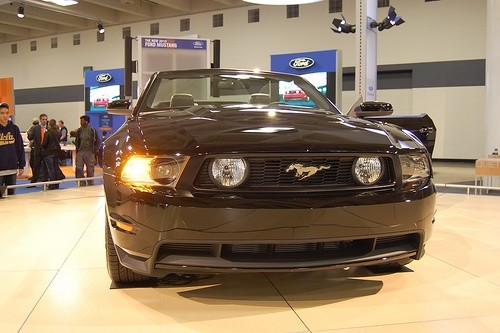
0 102 9 108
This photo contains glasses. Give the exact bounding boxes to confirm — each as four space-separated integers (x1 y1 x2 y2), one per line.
43 119 47 120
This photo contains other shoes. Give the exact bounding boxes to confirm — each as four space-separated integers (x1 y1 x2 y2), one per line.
26 177 37 188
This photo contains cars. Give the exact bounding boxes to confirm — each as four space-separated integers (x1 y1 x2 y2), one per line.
102 69 437 283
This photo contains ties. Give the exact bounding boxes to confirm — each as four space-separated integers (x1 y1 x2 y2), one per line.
41 125 45 144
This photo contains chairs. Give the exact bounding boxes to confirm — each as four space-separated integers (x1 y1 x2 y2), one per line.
249 93 271 105
169 93 194 108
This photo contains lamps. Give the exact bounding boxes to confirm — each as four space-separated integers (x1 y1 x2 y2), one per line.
371 5 405 31
96 21 109 34
331 13 356 34
17 6 25 19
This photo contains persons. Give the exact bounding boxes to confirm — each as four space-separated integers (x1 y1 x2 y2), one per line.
42 119 62 190
0 102 26 197
74 115 100 186
58 120 67 166
100 131 111 141
27 118 39 180
26 114 48 188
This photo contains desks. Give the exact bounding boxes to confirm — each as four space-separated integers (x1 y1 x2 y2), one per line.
60 146 76 171
475 159 500 195
